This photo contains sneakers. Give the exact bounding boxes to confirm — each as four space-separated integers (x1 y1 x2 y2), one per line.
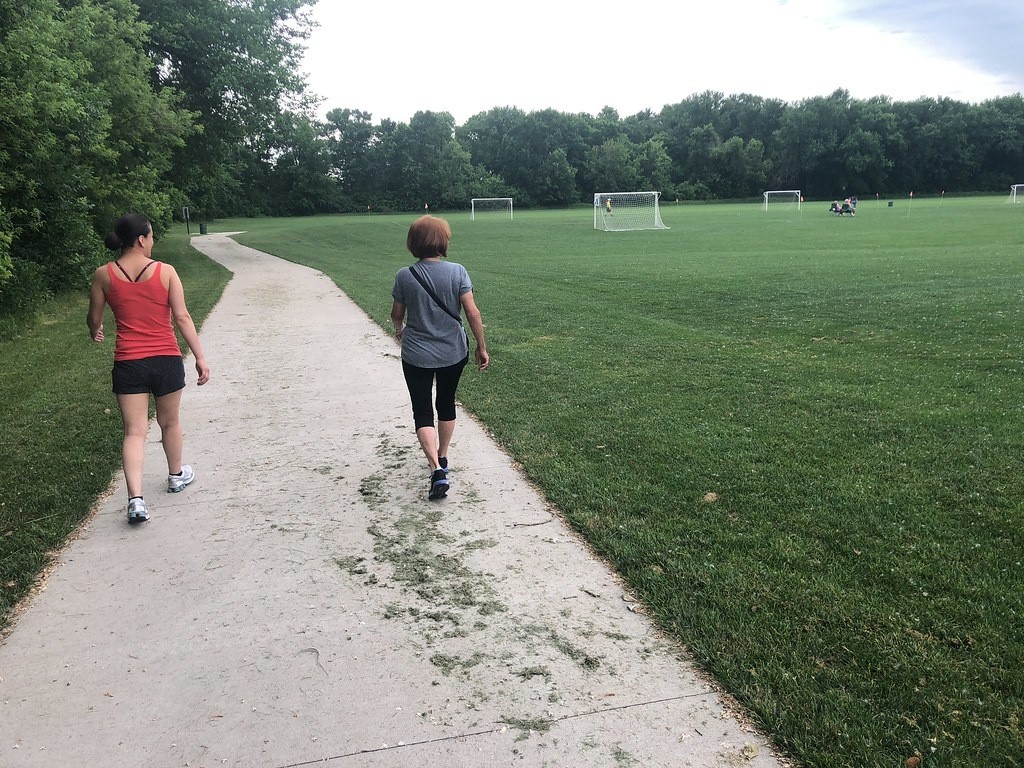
428 470 450 499
167 465 194 493
127 498 149 523
437 449 448 473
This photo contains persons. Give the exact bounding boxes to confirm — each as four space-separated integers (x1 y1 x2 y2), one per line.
604 198 614 217
829 195 857 216
86 214 210 522
390 215 489 498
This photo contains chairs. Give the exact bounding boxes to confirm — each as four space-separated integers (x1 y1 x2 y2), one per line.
841 204 853 217
831 203 840 217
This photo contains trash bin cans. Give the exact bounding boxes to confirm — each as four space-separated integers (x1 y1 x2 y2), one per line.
888 202 893 207
199 224 208 234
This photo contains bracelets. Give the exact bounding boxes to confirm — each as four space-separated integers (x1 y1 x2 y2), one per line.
394 322 405 332
